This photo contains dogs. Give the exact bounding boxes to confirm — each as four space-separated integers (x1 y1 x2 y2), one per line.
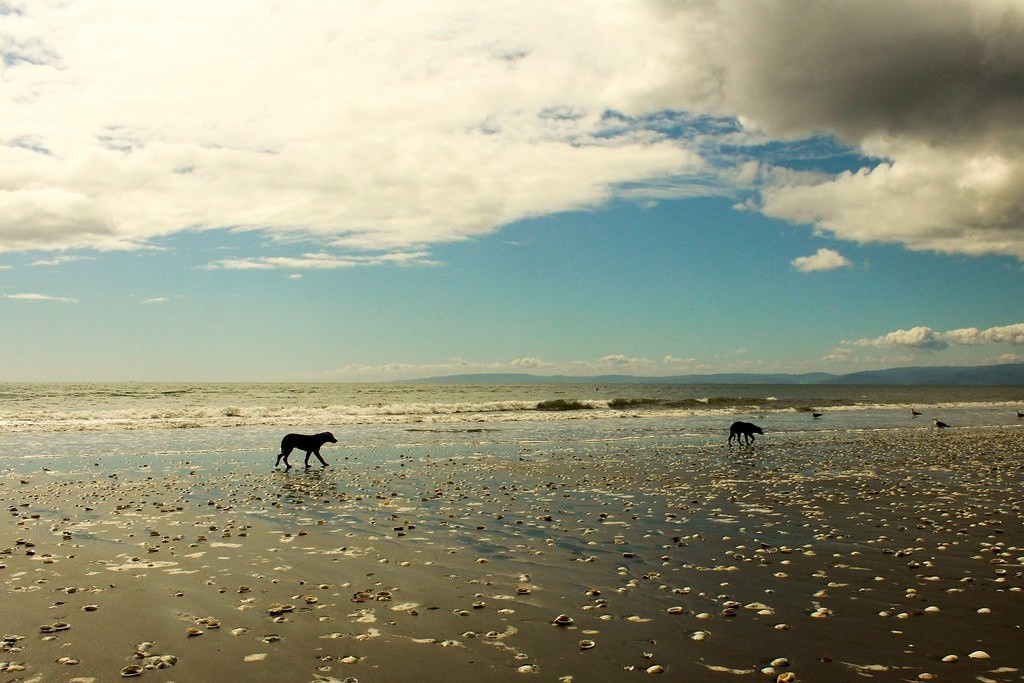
727 421 764 447
274 431 337 473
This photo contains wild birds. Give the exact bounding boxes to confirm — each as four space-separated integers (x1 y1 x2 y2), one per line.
811 409 823 419
910 408 922 418
932 417 951 433
1015 409 1024 420
